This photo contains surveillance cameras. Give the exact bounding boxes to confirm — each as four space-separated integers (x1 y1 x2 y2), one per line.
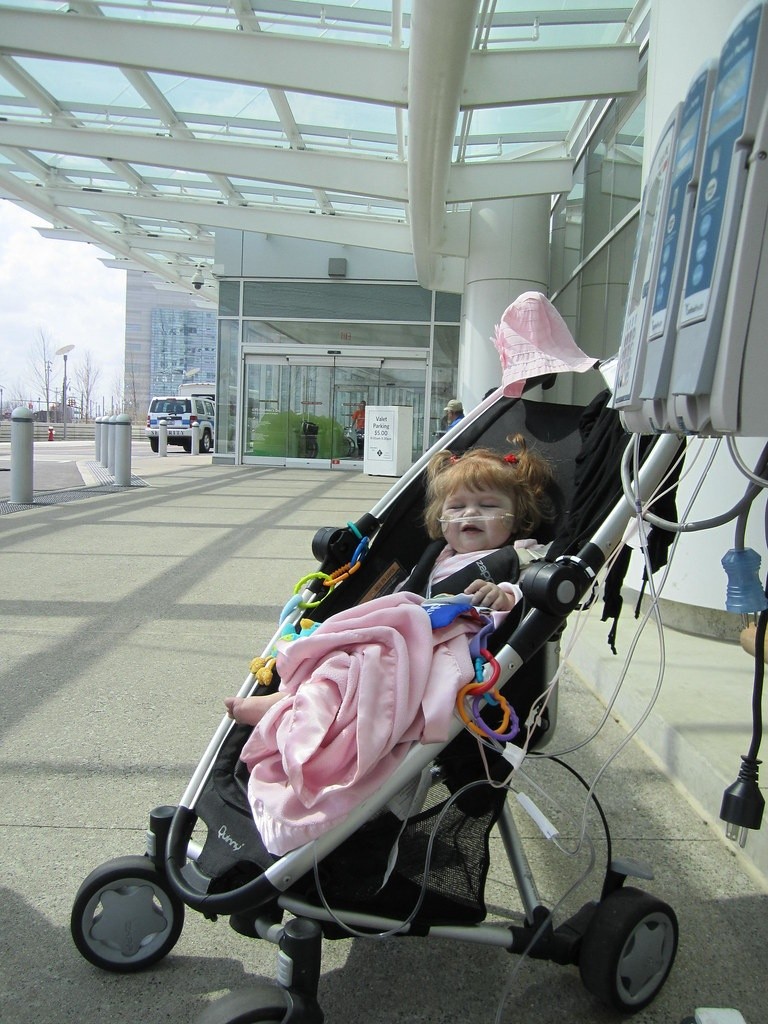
191 274 204 289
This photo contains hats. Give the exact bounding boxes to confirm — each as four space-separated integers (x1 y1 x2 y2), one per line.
489 291 600 397
443 399 462 410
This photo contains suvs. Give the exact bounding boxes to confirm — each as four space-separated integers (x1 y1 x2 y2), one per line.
146 396 217 452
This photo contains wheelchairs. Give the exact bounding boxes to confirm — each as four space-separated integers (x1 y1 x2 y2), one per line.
294 422 319 458
342 425 355 455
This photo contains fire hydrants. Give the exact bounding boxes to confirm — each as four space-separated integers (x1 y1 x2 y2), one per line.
48 427 56 442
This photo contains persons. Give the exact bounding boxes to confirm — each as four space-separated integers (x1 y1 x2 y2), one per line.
443 399 464 430
350 400 366 459
224 434 559 728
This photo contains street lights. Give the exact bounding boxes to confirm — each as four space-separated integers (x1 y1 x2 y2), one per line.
54 344 75 440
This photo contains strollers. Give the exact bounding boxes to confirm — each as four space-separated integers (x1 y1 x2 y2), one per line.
69 378 686 1023
